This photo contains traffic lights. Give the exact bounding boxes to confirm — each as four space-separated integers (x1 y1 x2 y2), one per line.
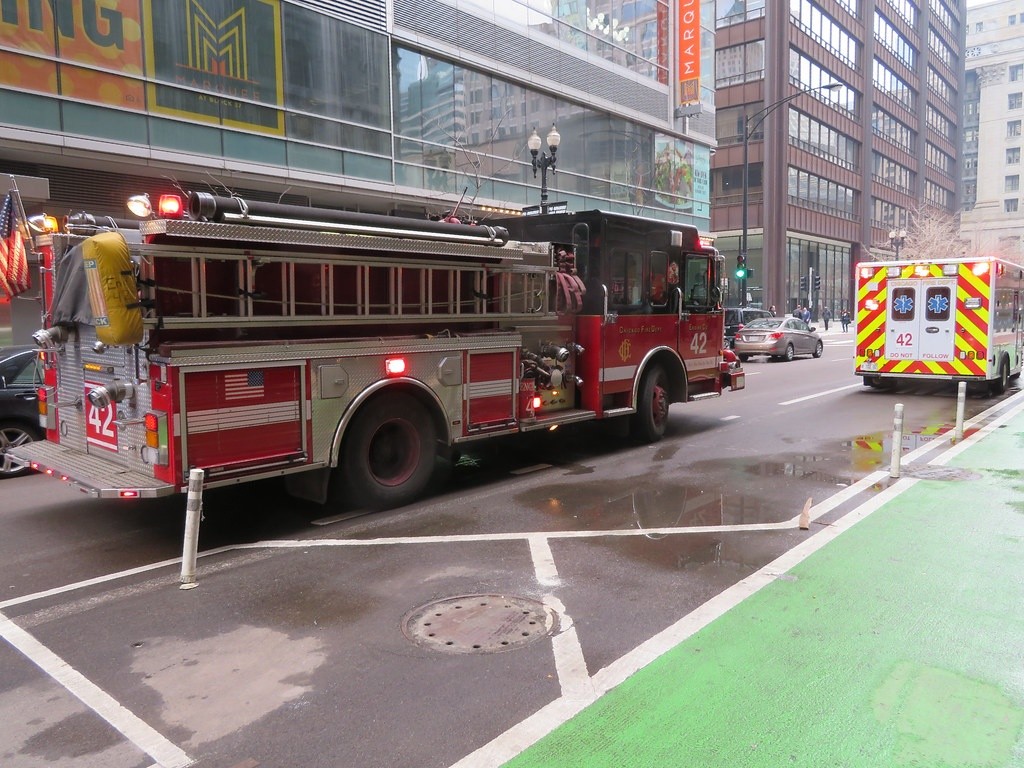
812 275 821 293
735 252 747 280
800 276 809 292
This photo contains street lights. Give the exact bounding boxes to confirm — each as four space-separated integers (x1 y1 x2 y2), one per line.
522 123 568 215
739 81 844 311
889 231 906 261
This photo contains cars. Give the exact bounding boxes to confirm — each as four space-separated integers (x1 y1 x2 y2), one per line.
734 317 824 362
0 344 45 480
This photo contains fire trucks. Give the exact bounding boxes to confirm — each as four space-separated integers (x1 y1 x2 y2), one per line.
4 190 746 519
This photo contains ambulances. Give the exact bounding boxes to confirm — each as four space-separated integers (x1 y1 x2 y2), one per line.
851 256 1024 396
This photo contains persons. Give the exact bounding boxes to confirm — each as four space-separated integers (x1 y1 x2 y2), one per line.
769 305 776 317
793 304 810 324
822 307 830 330
841 308 852 333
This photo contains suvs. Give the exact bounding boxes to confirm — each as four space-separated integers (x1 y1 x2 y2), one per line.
725 307 778 350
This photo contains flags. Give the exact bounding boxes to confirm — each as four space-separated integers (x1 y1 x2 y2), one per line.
0 192 32 302
